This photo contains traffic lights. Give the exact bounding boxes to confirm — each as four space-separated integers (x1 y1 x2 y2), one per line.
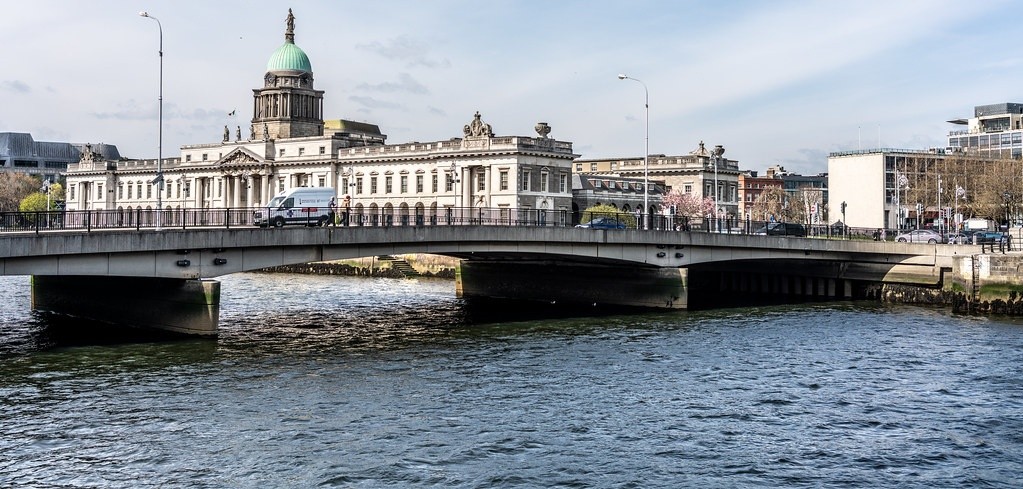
841 203 843 212
951 208 954 217
921 204 924 214
940 208 946 218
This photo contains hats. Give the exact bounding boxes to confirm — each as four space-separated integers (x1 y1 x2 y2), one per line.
330 196 334 201
346 196 350 199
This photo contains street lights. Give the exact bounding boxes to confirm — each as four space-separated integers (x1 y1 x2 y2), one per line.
897 175 910 235
708 154 720 233
241 171 251 226
618 74 649 230
449 163 457 225
956 188 967 245
346 166 356 225
141 12 164 227
41 180 52 228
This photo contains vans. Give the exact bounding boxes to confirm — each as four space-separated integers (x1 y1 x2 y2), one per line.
254 187 337 229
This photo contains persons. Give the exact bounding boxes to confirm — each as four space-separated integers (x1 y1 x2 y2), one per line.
769 214 775 221
873 229 881 241
341 195 353 226
325 196 337 226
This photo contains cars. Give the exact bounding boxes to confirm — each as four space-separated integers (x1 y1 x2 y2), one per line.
574 218 626 229
755 223 804 236
895 219 1008 245
